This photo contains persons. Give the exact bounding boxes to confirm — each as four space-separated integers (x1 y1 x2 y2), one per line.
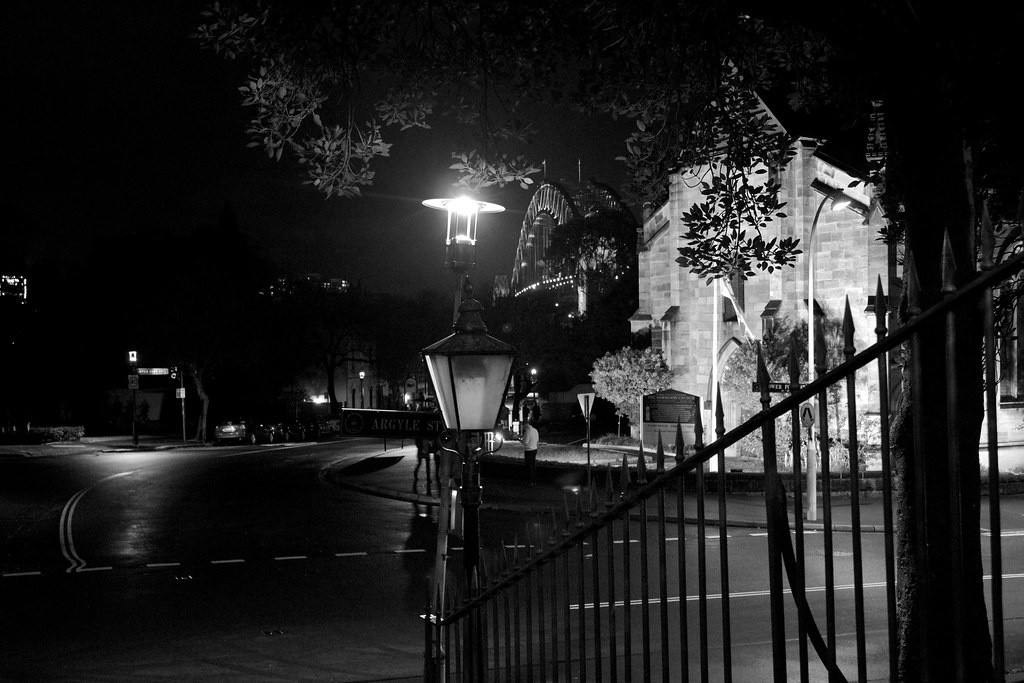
413 438 433 484
519 422 539 487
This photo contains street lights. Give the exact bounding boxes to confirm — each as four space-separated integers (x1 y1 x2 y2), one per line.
418 189 508 496
803 185 851 521
359 370 366 409
